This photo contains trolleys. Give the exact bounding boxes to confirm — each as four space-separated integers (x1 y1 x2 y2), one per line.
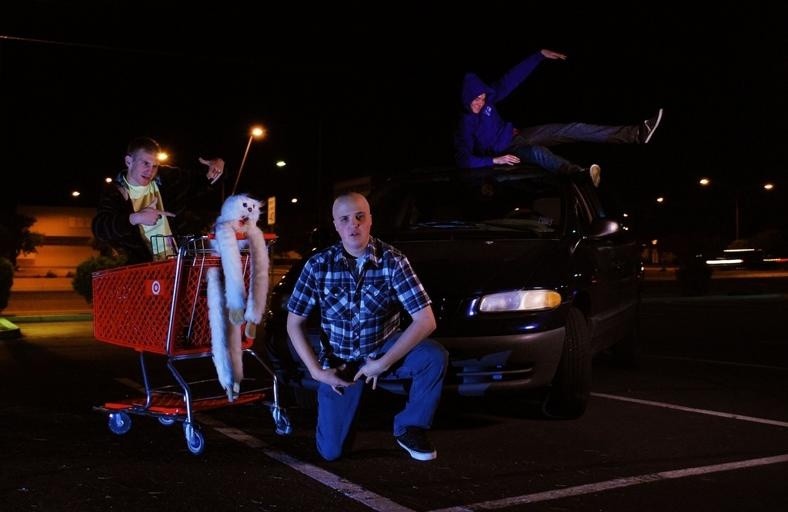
87 225 295 458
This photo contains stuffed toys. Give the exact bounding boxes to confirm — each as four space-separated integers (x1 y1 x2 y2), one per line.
201 191 270 404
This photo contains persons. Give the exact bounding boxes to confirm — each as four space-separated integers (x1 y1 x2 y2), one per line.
452 48 666 184
285 192 453 466
89 138 230 260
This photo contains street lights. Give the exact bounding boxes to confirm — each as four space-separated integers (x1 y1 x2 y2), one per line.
696 176 775 241
231 120 272 192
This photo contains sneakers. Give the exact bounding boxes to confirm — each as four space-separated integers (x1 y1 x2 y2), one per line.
639 108 664 143
589 164 601 187
397 426 437 461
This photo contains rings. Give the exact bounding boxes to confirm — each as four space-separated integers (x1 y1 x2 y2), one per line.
217 169 220 174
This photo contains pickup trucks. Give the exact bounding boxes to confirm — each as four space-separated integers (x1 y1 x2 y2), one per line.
262 159 648 423
